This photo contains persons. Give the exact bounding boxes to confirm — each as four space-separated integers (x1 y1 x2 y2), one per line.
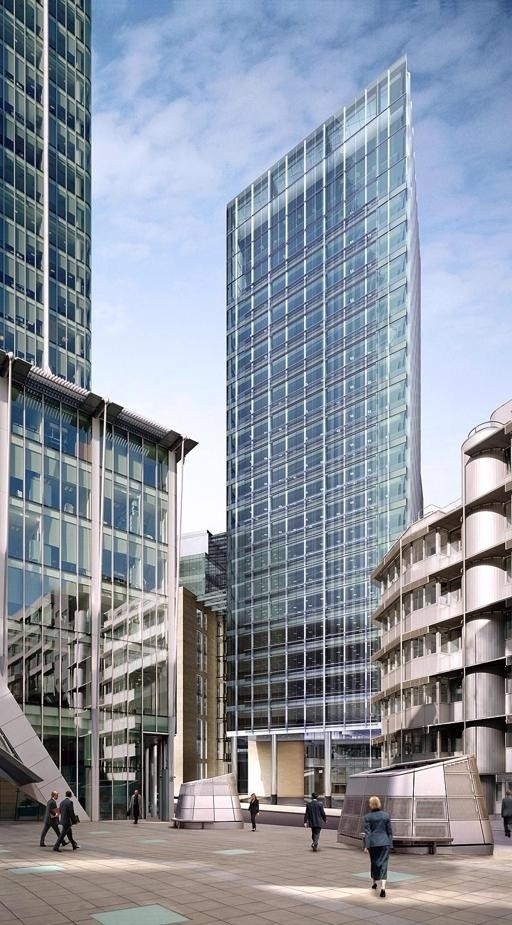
304 793 326 852
53 791 79 852
501 790 512 837
40 790 70 847
363 796 392 897
128 790 142 824
248 793 259 831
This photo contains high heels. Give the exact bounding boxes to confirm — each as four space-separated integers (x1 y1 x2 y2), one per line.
379 888 385 897
371 882 377 889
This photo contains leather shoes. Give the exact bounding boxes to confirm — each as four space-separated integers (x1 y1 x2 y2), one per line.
40 841 70 848
53 845 81 852
251 827 256 831
311 843 317 851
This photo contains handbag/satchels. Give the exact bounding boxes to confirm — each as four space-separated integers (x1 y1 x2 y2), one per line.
49 807 61 819
69 815 80 826
